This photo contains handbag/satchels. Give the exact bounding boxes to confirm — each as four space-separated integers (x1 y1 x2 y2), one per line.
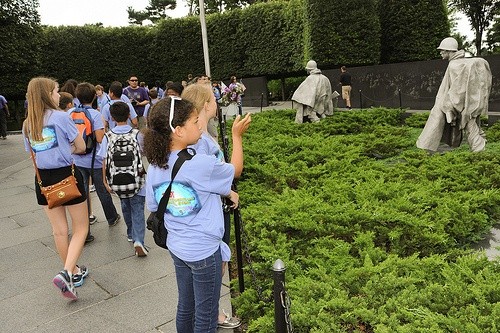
147 214 168 248
38 176 82 209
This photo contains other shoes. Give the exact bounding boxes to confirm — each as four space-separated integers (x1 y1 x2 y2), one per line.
218 317 242 329
85 236 95 243
345 106 353 111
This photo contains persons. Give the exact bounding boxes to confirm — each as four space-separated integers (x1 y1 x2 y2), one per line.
290 60 334 123
64 81 122 243
181 83 242 329
22 77 92 302
145 96 252 333
417 36 487 155
24 74 247 192
94 102 149 257
339 66 353 110
0 94 11 138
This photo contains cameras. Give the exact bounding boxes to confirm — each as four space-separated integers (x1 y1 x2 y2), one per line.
223 198 234 215
131 99 137 106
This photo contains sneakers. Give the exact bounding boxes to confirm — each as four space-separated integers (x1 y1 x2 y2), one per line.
127 236 133 241
73 264 89 287
53 270 78 299
134 241 149 256
89 215 99 224
109 214 120 226
89 184 96 193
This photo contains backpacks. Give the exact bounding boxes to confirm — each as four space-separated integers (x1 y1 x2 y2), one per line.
105 128 147 199
69 105 98 156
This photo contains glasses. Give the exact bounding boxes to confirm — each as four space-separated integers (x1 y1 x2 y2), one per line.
169 94 182 133
129 79 139 82
